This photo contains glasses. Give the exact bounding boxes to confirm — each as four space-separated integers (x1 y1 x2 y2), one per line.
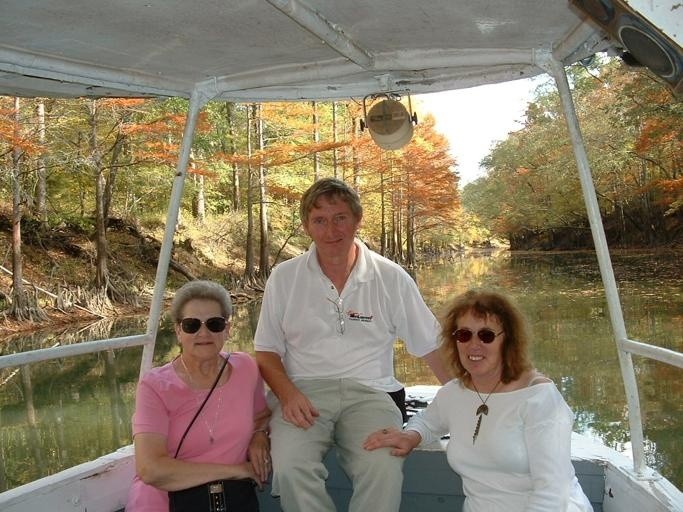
448 329 505 344
326 297 344 334
174 318 226 334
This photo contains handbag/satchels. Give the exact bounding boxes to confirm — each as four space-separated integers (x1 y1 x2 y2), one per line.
168 476 259 510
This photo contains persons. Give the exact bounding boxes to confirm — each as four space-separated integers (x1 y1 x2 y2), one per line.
363 288 595 512
255 177 459 511
120 280 273 512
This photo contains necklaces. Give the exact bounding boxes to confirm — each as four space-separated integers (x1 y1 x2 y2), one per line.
180 352 222 444
460 377 510 442
